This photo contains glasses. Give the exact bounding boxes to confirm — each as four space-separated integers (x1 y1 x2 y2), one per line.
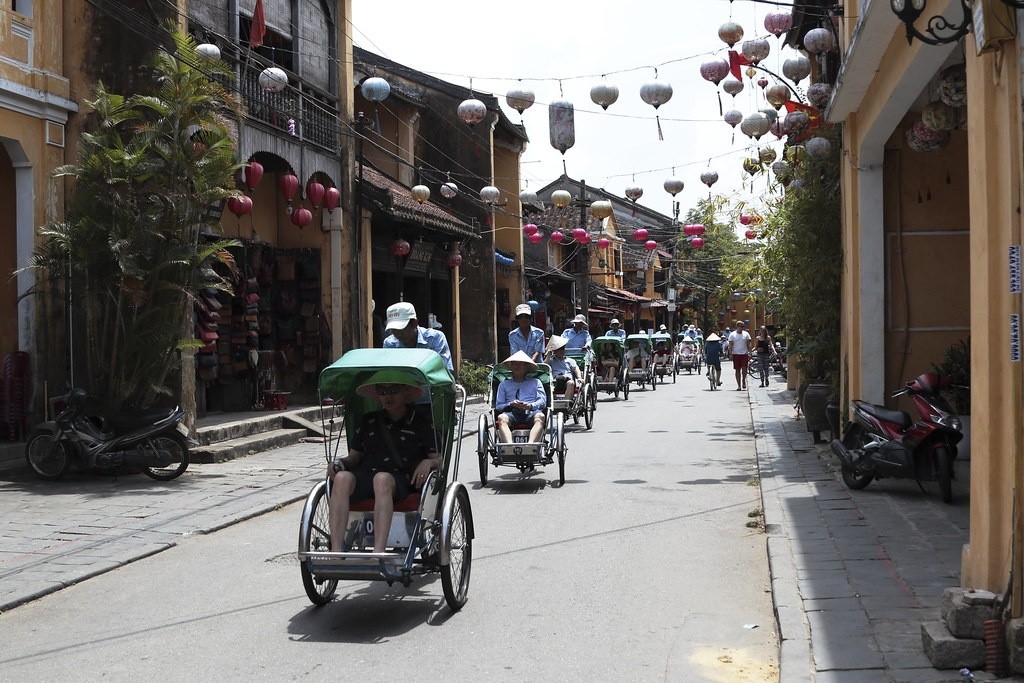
517 314 531 319
376 384 403 396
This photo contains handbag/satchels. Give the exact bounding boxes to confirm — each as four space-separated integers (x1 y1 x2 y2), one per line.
603 358 619 368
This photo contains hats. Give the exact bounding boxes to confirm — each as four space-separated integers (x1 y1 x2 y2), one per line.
384 302 418 332
688 325 696 330
501 350 539 371
683 336 693 342
546 334 569 351
724 327 730 330
570 314 588 326
706 333 721 341
682 324 689 331
659 324 666 330
355 370 426 403
609 319 622 328
515 304 532 316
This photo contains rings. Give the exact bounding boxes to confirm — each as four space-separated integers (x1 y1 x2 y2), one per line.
421 475 424 477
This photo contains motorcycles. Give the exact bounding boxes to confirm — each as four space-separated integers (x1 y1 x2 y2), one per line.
830 364 970 505
25 377 200 482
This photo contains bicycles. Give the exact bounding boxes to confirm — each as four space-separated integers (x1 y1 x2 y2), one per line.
746 341 788 380
704 352 724 392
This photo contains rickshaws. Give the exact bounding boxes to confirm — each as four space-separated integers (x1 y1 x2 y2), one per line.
675 333 706 375
547 347 598 429
720 337 734 361
476 360 570 485
592 335 630 400
626 333 657 391
297 344 475 611
649 333 677 384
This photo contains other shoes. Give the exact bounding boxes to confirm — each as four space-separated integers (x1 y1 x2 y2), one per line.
717 382 720 386
742 385 746 388
706 372 710 376
759 384 764 387
765 379 769 387
737 388 741 391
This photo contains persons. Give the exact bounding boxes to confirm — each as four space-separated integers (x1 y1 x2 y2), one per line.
496 350 547 443
544 302 735 560
752 325 777 388
383 302 454 372
728 320 751 390
509 304 546 363
325 368 439 560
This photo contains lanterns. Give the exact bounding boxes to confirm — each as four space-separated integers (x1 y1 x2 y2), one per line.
191 11 833 265
